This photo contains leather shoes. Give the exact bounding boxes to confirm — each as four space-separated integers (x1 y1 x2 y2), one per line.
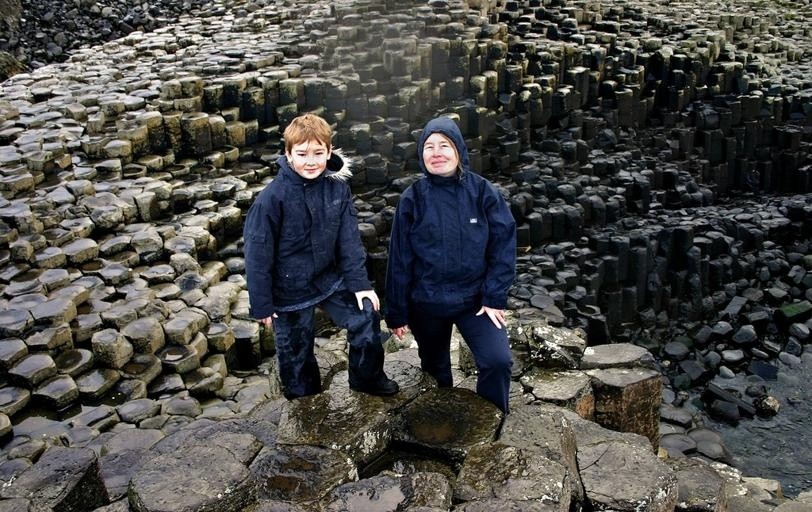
349 378 400 396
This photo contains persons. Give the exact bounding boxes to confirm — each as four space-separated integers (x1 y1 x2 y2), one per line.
244 113 400 401
383 117 517 414
